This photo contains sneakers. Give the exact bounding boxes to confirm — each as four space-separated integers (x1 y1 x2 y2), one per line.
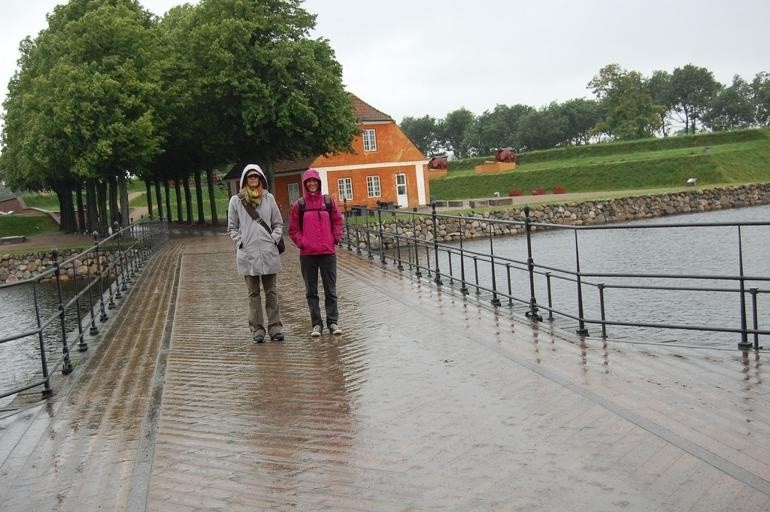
328 324 342 335
271 333 284 341
311 325 322 337
252 333 264 343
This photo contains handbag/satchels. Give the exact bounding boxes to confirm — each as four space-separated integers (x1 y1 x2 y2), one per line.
275 238 285 254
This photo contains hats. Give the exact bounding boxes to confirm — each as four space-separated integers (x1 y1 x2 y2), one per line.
247 170 261 177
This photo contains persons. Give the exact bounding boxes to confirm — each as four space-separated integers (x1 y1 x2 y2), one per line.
288 168 344 337
227 164 285 343
